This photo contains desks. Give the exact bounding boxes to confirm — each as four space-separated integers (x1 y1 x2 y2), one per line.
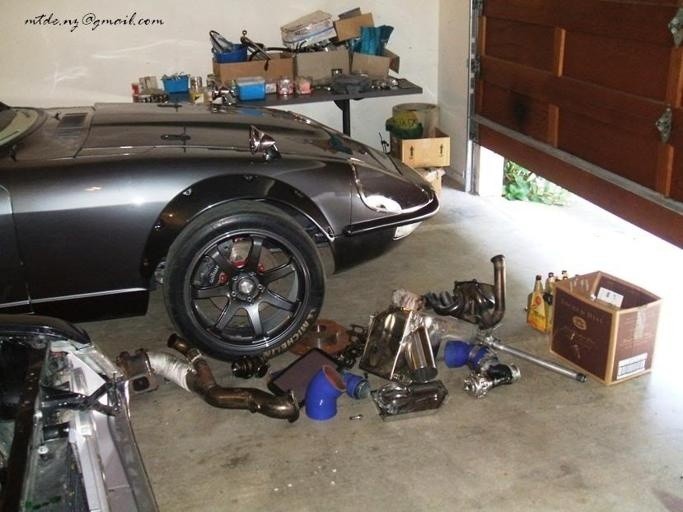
140 76 421 139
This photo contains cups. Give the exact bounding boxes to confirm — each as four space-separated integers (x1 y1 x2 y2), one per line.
331 69 342 75
189 77 202 94
294 76 312 95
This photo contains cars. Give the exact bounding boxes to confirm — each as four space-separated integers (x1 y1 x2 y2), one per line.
0 94 439 364
0 315 160 512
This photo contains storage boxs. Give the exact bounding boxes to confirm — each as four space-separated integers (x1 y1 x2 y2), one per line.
391 129 451 171
212 8 400 87
237 75 265 101
420 168 442 199
551 269 659 386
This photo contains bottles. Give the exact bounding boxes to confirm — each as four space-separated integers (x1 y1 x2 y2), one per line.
277 73 293 95
527 270 568 333
